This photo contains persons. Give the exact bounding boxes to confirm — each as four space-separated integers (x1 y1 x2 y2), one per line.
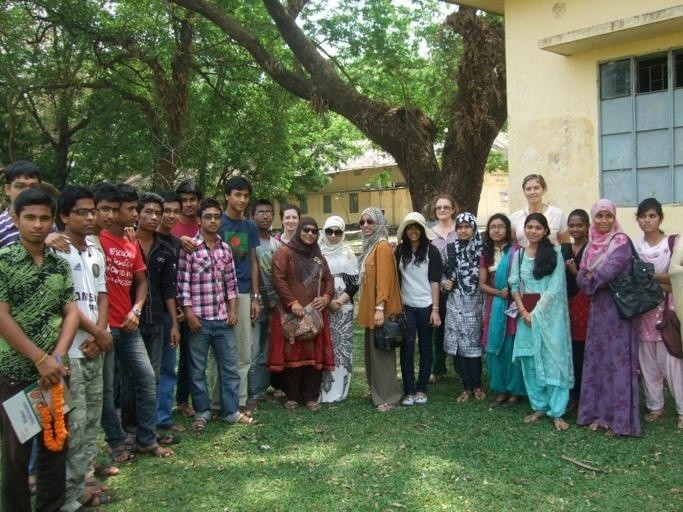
479 213 525 409
317 216 360 403
576 198 642 439
422 195 459 383
1 161 263 512
271 216 335 412
508 174 570 243
357 207 405 413
246 198 283 408
632 198 683 429
508 212 570 431
274 204 300 245
561 209 591 414
439 211 488 403
393 212 442 407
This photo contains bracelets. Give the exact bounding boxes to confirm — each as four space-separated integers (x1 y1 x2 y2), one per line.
520 308 526 314
431 307 439 309
374 306 384 310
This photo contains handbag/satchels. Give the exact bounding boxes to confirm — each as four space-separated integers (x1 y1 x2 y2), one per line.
280 302 325 345
440 290 448 331
607 233 664 319
374 312 414 352
656 312 683 358
504 280 525 318
266 311 273 335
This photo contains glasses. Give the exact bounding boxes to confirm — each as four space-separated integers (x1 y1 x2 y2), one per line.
202 213 221 220
408 227 419 232
435 205 452 210
70 207 100 216
489 225 506 229
14 181 40 190
302 218 373 237
254 211 273 217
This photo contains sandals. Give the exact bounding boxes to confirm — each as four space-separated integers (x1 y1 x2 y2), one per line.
85 432 174 505
181 391 322 426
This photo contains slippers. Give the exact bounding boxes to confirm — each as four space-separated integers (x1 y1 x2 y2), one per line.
677 416 683 430
29 482 37 495
456 390 570 429
644 410 667 424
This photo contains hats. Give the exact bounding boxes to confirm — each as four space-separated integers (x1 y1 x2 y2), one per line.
397 212 438 241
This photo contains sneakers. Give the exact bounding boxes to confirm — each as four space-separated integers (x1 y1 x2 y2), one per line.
375 389 428 411
